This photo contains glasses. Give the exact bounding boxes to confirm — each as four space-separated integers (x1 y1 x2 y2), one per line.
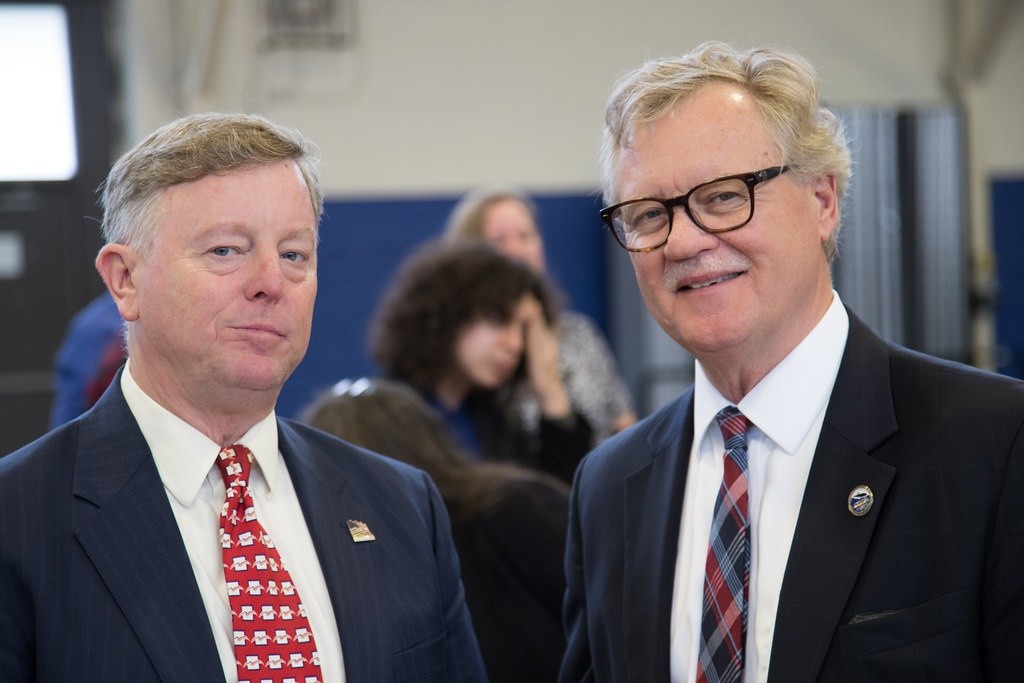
599 164 795 253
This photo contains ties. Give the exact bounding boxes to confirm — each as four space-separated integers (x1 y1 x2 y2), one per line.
216 444 324 683
696 404 754 683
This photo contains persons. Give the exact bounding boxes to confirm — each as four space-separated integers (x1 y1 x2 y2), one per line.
50 183 638 683
0 112 484 683
555 45 1024 683
